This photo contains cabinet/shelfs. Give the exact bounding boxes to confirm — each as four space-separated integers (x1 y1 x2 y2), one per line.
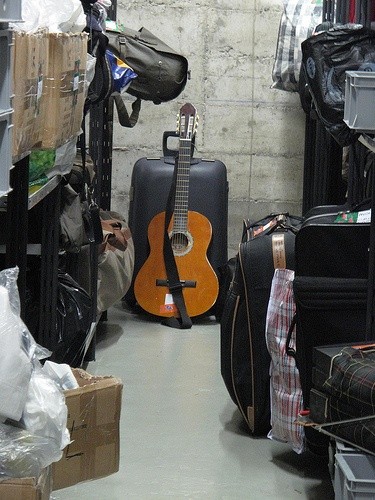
0 0 119 373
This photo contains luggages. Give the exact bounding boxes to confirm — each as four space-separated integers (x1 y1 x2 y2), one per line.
127 131 230 322
220 212 307 440
283 203 375 453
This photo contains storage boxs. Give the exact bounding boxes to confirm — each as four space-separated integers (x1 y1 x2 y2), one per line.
0 367 124 500
343 70 375 130
328 438 375 500
10 28 90 160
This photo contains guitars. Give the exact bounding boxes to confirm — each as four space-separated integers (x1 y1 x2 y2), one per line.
134 103 219 318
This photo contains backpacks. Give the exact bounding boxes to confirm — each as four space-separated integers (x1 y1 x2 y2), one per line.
104 21 189 127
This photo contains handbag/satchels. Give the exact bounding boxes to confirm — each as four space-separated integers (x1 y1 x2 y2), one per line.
265 266 308 455
75 208 134 313
270 0 322 92
